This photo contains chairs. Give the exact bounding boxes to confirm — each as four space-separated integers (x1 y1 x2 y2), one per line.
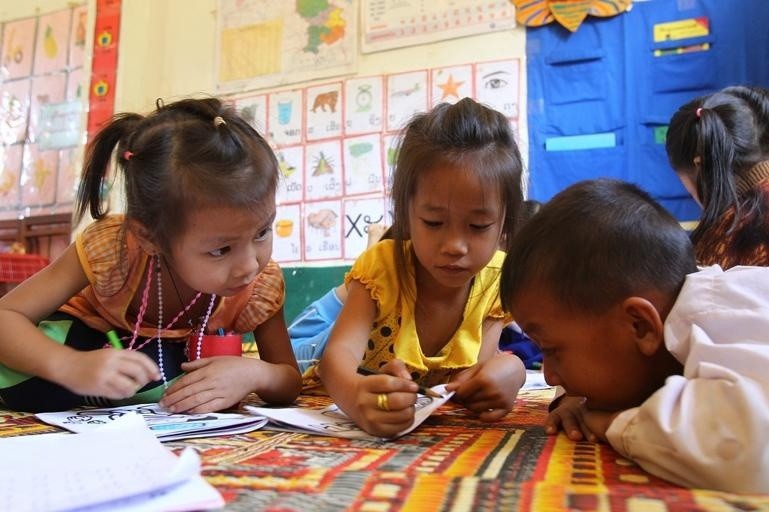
0 219 22 245
20 210 72 255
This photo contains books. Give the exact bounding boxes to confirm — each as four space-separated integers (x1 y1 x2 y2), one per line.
32 401 268 444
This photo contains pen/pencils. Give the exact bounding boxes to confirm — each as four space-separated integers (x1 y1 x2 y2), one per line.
106 329 124 350
357 366 444 398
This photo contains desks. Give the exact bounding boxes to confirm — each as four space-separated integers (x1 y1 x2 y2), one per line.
0 249 51 300
0 375 769 512
